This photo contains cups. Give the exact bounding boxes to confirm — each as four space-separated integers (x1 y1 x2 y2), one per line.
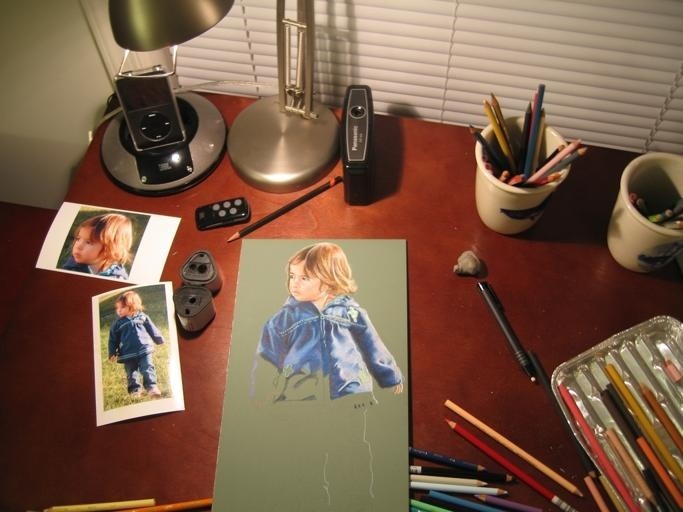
605 151 683 277
473 114 572 237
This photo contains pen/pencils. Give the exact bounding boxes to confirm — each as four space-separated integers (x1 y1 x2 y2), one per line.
445 416 575 512
468 83 589 188
43 498 214 512
524 348 595 479
583 475 608 512
629 192 683 230
558 363 683 512
446 399 584 496
227 175 343 242
478 281 535 386
407 448 547 511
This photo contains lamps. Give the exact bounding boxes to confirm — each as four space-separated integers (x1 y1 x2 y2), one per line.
103 0 340 197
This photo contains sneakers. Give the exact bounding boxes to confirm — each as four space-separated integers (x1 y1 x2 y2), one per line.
131 392 140 397
148 388 161 396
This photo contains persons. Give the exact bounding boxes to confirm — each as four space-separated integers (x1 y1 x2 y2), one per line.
108 291 165 397
62 213 132 279
249 241 404 401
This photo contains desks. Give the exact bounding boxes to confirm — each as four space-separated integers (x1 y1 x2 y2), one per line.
0 78 683 512
